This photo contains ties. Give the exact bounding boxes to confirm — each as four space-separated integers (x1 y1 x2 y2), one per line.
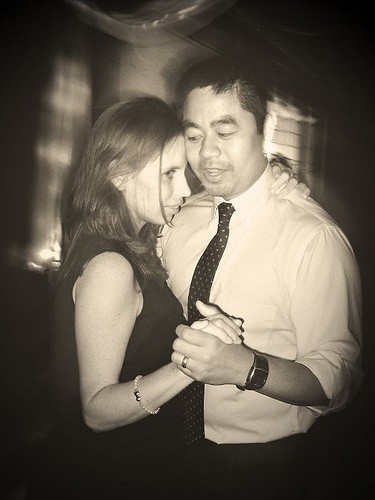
178 203 235 449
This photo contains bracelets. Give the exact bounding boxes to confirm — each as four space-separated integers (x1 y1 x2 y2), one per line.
134 375 160 415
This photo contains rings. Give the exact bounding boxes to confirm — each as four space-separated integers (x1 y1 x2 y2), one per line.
182 356 189 368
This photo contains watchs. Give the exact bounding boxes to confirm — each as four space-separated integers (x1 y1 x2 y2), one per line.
236 350 269 391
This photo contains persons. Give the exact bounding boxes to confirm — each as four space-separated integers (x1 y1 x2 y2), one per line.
155 56 365 500
54 94 244 500
